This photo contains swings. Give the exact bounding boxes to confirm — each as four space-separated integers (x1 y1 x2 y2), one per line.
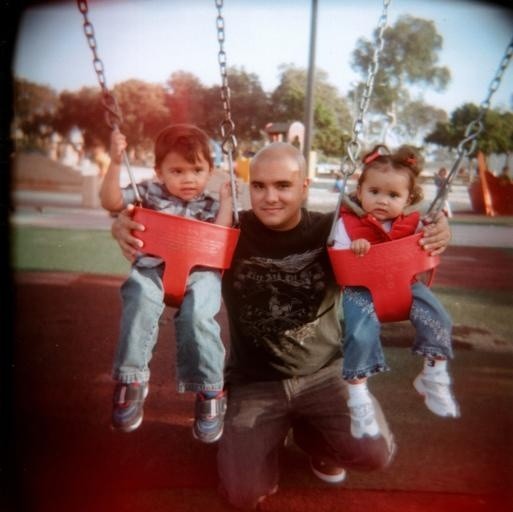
77 0 241 308
328 1 513 322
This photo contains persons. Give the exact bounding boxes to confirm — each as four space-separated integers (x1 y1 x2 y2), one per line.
110 141 453 510
500 166 512 183
98 124 242 444
332 170 344 193
433 167 451 218
330 145 462 439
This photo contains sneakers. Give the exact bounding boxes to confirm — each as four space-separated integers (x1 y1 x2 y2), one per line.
311 456 347 483
110 382 149 433
347 396 378 440
192 389 228 443
413 369 456 418
